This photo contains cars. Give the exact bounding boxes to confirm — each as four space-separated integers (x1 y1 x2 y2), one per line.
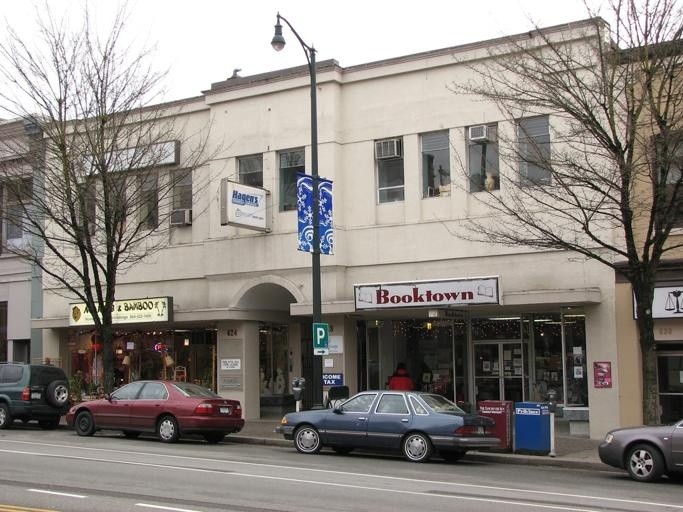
271 388 502 462
598 418 683 482
65 380 244 442
449 373 499 399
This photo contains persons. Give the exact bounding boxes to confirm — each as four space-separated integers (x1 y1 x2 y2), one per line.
387 368 415 391
43 357 53 366
69 368 88 396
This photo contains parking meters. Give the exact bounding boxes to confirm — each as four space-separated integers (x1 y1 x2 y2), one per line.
547 390 558 457
291 377 305 412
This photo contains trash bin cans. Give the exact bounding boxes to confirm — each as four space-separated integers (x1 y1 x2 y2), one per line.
479 400 513 453
514 401 550 455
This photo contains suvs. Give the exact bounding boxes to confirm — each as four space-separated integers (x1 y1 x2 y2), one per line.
0 361 68 430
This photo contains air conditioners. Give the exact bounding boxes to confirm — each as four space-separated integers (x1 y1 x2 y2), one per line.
468 125 489 142
169 209 192 225
375 139 400 159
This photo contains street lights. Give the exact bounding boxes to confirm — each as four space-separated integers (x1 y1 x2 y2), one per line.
269 11 325 411
